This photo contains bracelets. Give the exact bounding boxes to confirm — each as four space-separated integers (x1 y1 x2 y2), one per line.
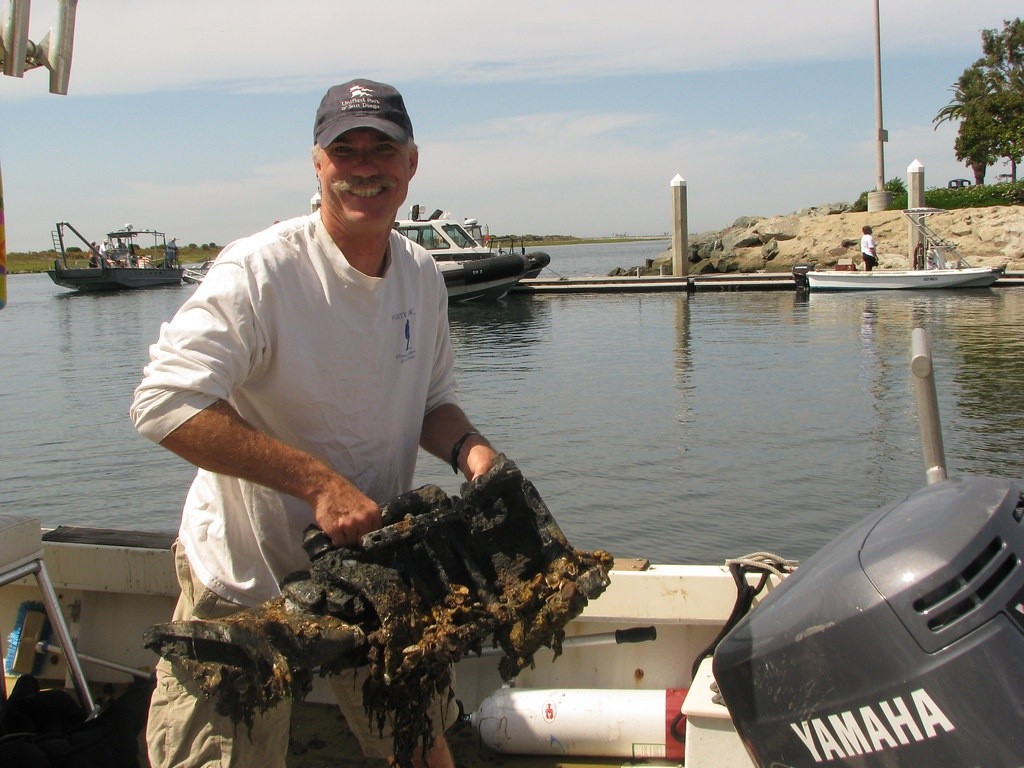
451 432 479 476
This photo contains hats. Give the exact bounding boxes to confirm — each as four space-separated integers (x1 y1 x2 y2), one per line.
104 239 109 242
313 78 414 149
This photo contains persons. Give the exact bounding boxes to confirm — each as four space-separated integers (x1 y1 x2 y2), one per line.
99 240 112 269
167 237 178 269
89 241 99 269
861 225 879 271
129 79 499 768
115 239 127 248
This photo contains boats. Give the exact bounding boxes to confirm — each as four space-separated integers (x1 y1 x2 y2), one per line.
42 222 186 290
803 209 1008 291
179 198 553 310
